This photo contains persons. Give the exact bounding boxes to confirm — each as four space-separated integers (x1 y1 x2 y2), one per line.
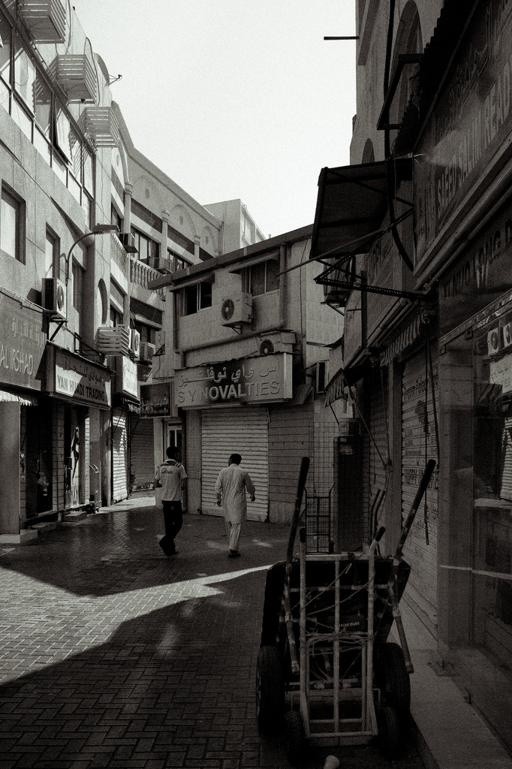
214 454 256 557
153 446 188 556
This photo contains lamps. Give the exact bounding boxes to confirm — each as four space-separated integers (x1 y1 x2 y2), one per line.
65 223 120 276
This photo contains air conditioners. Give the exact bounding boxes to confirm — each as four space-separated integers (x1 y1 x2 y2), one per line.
40 277 66 320
131 329 140 355
257 333 294 354
219 293 252 324
154 256 171 271
140 342 156 363
121 232 135 247
487 323 512 356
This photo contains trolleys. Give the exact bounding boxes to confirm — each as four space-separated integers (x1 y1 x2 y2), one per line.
279 526 409 769
255 457 437 769
